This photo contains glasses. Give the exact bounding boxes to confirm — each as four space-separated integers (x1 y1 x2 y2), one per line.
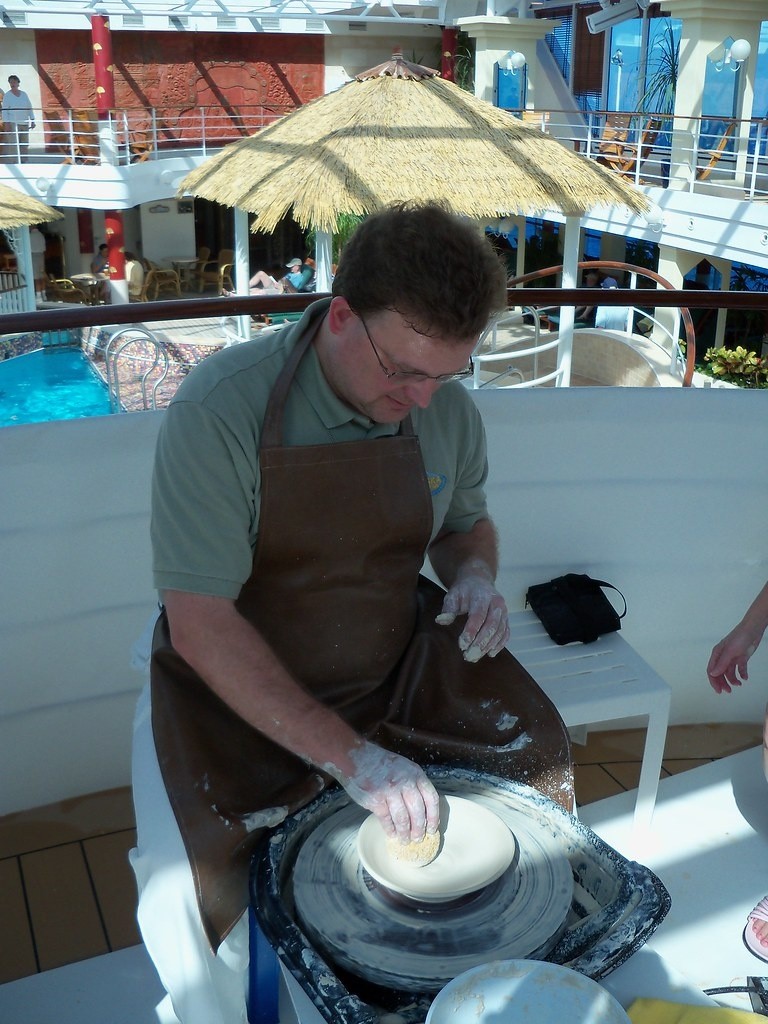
352 304 475 385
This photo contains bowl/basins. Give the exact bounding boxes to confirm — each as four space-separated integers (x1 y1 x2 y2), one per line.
356 795 515 903
424 959 633 1024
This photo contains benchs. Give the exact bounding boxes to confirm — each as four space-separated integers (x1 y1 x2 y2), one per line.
497 609 674 816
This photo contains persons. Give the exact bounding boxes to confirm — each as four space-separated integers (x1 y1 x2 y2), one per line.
90 243 108 273
575 274 600 320
128 199 579 1024
2 75 36 164
706 582 768 964
594 277 629 331
218 258 303 298
125 251 144 297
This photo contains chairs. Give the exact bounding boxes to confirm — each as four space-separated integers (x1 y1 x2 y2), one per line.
593 113 736 188
39 109 152 167
40 247 337 305
510 234 753 365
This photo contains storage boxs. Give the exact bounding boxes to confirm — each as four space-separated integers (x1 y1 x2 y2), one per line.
661 157 671 189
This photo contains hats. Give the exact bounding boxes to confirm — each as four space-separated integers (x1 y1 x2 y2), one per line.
585 273 598 279
286 258 303 268
601 277 618 289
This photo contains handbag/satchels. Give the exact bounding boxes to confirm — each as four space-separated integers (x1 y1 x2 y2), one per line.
528 574 627 645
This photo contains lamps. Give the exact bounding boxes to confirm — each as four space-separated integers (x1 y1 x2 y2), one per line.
586 0 653 34
708 39 751 72
497 51 525 76
490 218 514 240
645 205 666 234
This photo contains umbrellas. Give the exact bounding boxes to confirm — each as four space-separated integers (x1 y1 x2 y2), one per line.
0 182 65 230
181 46 652 234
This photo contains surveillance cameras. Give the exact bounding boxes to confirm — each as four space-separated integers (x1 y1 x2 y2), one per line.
585 0 640 35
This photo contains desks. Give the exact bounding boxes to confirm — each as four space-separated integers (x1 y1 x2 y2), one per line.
71 272 109 306
164 256 199 293
